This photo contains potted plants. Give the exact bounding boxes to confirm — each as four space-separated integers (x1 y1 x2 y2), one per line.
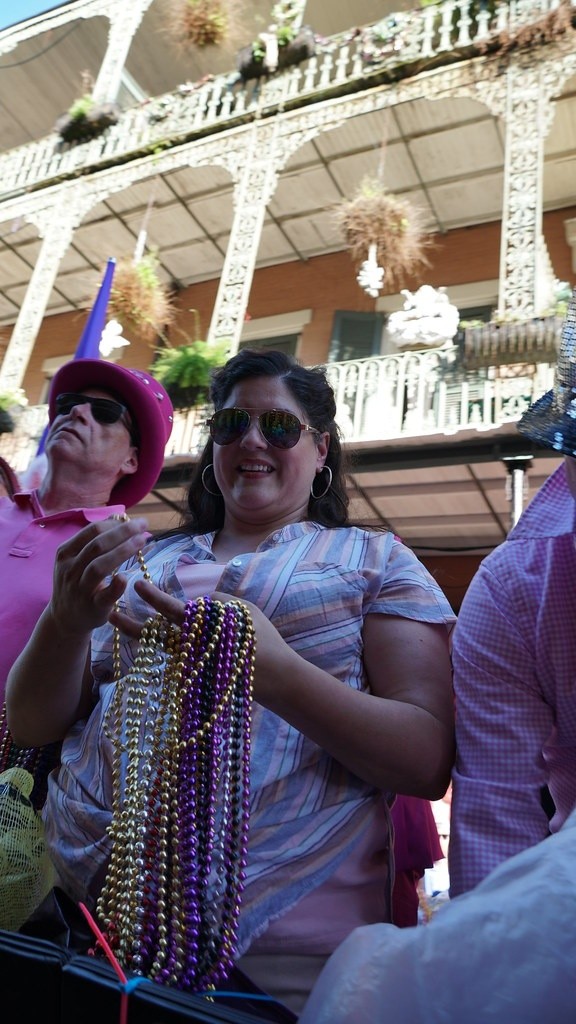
149 340 231 409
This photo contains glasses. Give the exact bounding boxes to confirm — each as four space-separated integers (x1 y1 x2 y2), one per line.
55 392 139 446
205 407 321 450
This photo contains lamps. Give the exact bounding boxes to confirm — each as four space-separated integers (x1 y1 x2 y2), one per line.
96 174 162 356
357 139 390 300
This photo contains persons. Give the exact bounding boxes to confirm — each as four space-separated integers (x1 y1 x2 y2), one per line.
389 793 446 926
0 359 173 767
5 352 457 1018
449 307 576 899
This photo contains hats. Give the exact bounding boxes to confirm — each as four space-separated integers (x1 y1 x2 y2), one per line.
515 287 575 455
47 357 173 509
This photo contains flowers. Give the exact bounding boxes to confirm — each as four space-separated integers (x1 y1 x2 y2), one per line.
251 1 310 71
71 95 93 120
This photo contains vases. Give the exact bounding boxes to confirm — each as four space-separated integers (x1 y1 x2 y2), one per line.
60 105 120 140
239 28 315 83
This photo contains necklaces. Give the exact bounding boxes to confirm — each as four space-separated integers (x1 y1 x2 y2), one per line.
0 701 43 779
96 514 255 1002
